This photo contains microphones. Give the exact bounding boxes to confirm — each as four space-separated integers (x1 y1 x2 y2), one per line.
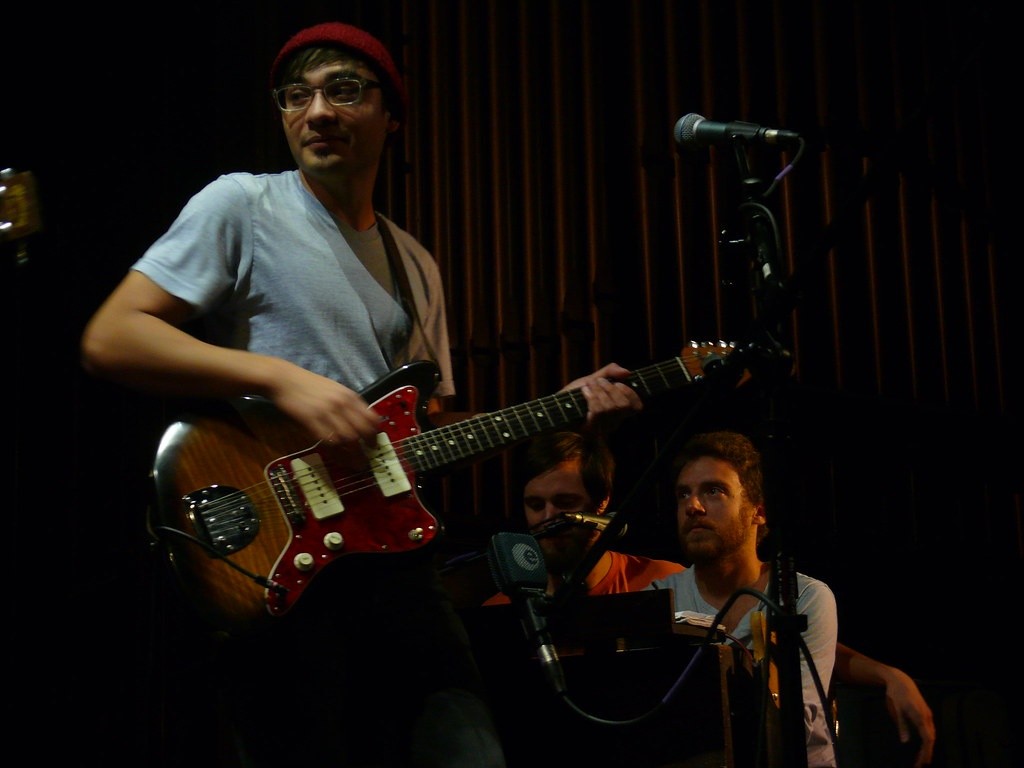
674 112 799 150
487 533 569 694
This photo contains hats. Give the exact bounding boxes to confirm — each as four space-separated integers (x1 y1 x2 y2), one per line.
269 21 412 121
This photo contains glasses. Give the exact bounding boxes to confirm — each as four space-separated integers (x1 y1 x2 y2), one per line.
272 72 387 113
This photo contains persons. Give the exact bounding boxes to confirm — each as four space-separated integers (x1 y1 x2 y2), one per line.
82 18 645 768
483 430 936 768
628 435 841 768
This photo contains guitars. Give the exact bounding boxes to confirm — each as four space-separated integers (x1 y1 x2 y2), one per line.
150 340 786 628
